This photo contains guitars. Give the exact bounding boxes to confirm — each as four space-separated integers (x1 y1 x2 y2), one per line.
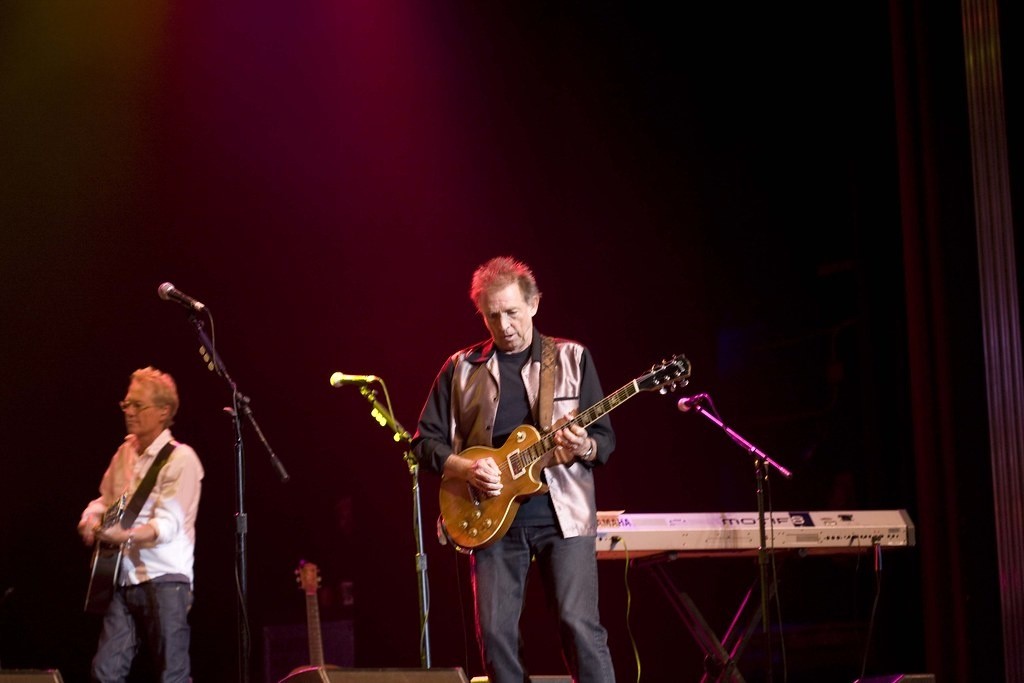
438 352 693 554
85 495 128 614
296 558 328 668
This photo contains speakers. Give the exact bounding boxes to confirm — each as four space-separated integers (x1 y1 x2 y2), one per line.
0 668 65 683
277 666 469 683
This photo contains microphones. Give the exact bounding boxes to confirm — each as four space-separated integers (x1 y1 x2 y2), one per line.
329 372 381 388
157 283 209 311
677 393 707 412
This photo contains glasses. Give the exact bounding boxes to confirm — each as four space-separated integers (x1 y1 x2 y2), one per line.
120 401 169 412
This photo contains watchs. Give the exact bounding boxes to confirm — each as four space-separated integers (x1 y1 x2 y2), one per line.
582 441 593 461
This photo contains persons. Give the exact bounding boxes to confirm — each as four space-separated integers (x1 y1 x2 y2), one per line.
77 367 204 683
409 257 616 683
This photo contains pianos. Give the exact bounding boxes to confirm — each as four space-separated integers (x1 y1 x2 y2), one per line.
596 508 916 561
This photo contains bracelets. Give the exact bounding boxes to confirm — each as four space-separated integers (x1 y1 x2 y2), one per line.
126 530 133 550
